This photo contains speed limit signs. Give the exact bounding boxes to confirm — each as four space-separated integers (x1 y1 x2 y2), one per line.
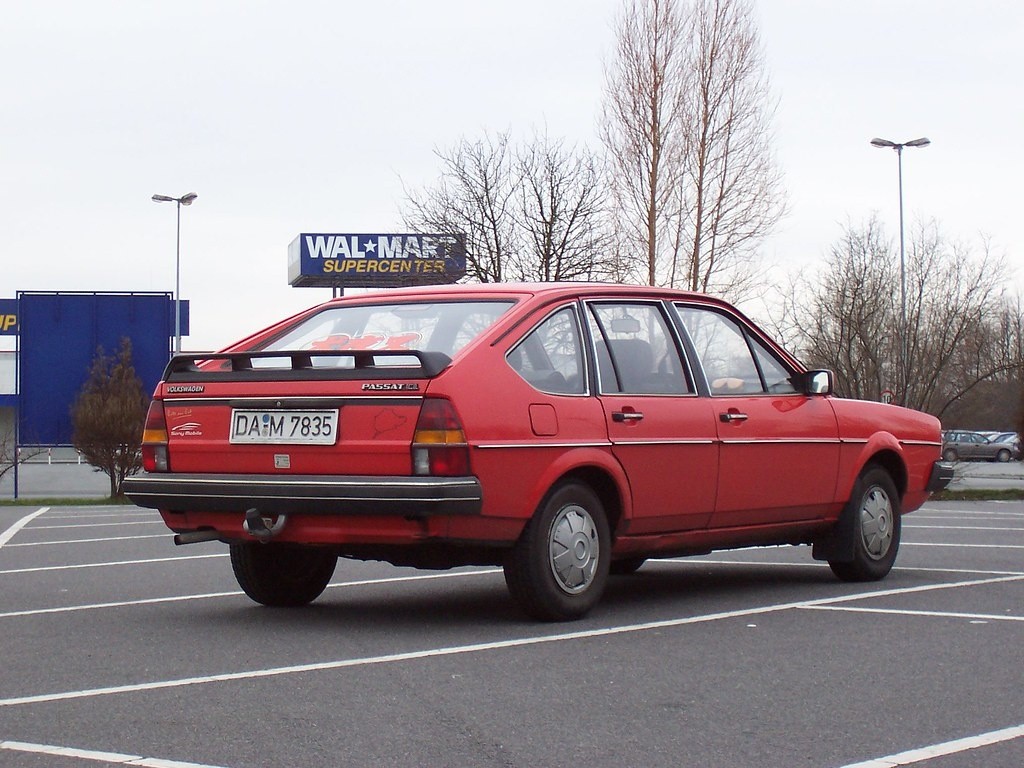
879 391 896 405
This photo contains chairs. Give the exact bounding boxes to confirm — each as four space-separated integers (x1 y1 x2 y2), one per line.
595 339 654 379
962 436 969 442
507 348 567 393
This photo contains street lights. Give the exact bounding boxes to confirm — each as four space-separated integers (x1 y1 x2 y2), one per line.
151 192 197 353
871 137 931 408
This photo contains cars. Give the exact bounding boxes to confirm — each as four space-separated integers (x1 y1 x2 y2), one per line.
973 431 1024 460
122 282 954 622
941 430 1014 463
